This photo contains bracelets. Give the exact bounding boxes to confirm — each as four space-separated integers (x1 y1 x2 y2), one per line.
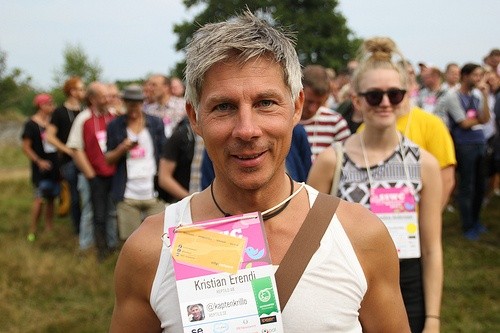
425 315 441 320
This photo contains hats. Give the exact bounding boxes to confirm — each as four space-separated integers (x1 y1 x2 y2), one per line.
121 85 146 100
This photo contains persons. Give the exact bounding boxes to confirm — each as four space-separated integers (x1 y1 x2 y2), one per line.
19 75 216 263
110 4 412 333
284 48 500 239
305 37 442 333
187 304 206 322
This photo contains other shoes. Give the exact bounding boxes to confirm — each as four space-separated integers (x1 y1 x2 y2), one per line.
462 222 487 240
26 232 37 244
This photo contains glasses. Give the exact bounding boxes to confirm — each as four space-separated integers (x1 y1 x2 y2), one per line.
358 88 406 107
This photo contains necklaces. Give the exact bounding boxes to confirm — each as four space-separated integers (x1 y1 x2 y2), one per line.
211 172 294 221
